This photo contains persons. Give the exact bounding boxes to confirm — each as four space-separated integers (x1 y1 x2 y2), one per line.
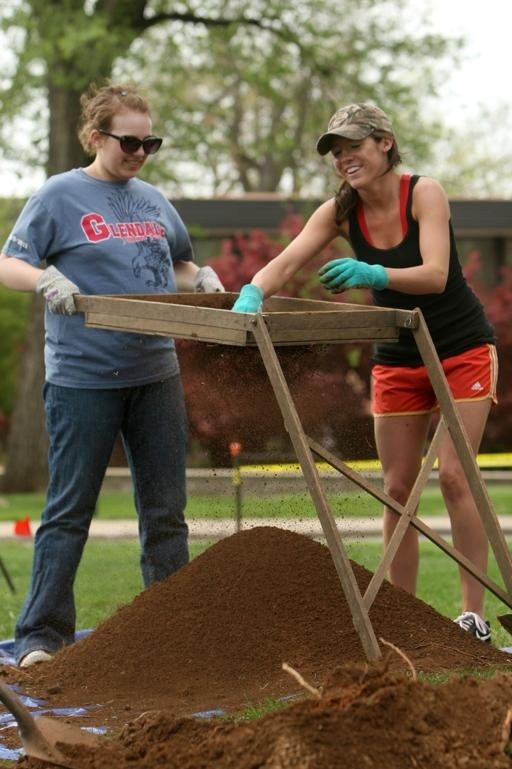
1 81 228 668
226 100 499 648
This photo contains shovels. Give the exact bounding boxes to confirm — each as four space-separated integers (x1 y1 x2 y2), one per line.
0 680 142 769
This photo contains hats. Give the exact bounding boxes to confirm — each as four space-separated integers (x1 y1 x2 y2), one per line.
316 102 393 155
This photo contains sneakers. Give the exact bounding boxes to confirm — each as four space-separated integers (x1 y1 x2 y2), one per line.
20 650 54 669
452 610 492 642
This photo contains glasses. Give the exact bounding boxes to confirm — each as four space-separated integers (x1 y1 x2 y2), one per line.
98 128 163 154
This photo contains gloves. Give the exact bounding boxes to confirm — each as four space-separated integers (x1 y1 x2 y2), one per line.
194 264 226 293
231 283 265 312
35 263 80 317
317 255 390 295
